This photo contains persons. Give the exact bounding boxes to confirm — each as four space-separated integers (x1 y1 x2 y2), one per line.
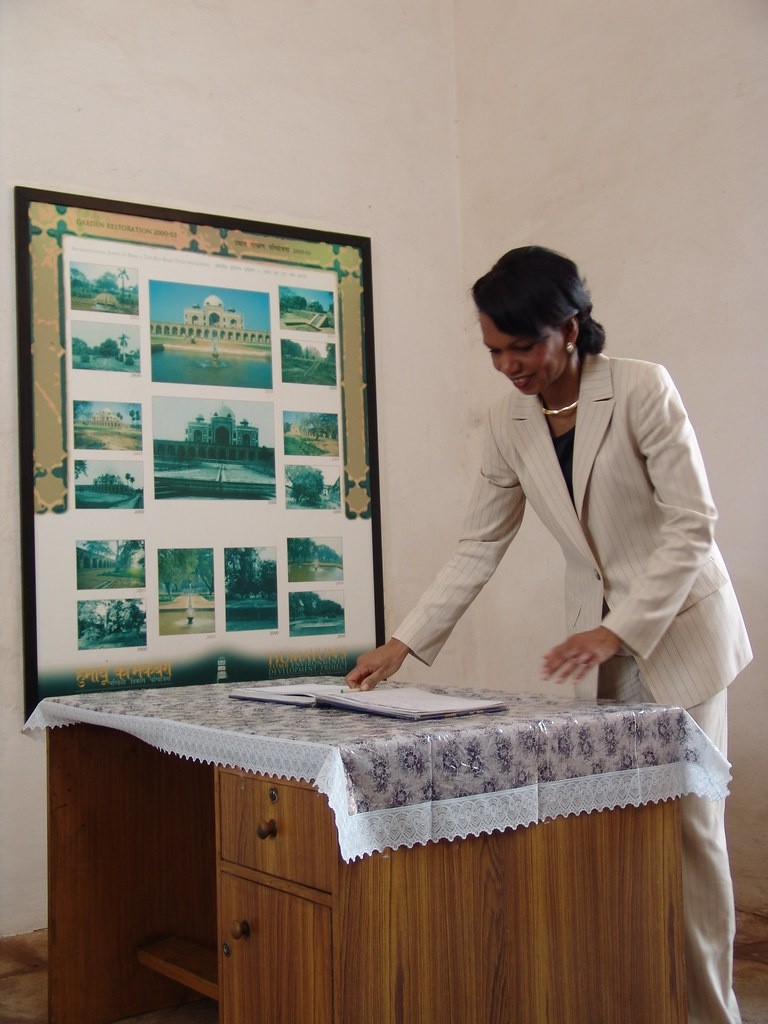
344 246 753 1024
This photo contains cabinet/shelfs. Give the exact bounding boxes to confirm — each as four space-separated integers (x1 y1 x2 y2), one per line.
20 676 734 1024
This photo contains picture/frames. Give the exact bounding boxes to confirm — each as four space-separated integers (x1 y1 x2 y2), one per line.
13 184 386 727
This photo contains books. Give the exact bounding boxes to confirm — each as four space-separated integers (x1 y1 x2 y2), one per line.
229 684 509 721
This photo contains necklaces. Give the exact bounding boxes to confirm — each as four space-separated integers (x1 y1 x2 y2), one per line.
542 400 579 415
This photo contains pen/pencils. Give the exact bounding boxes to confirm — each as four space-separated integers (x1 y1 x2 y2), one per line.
341 688 360 692
